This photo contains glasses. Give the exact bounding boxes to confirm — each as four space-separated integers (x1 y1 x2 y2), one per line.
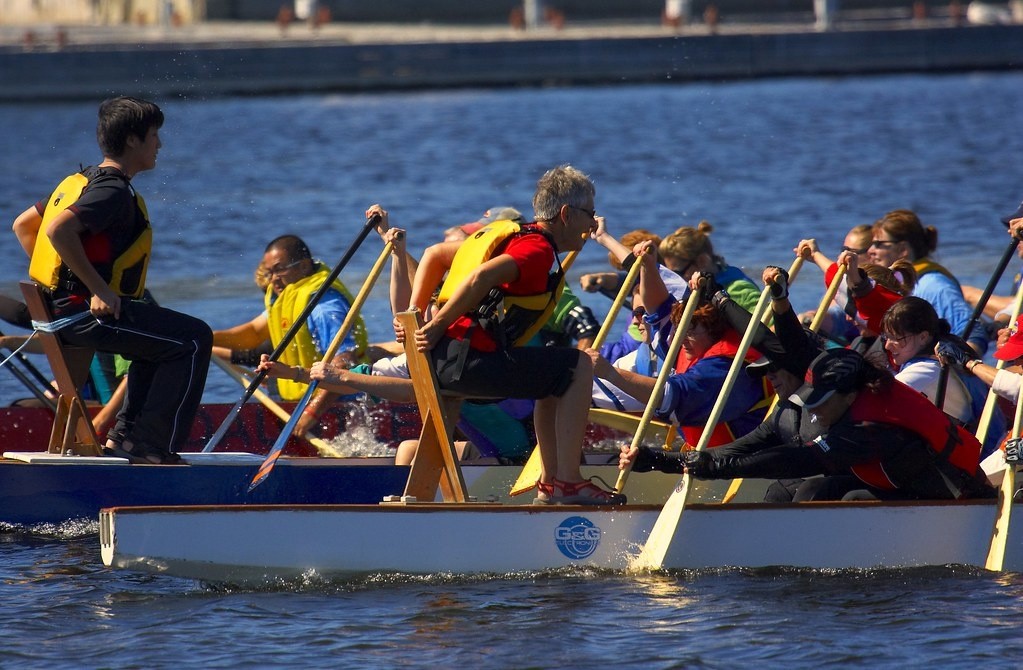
673 263 690 277
871 239 899 248
670 329 707 341
569 204 598 220
881 333 917 342
840 245 870 256
631 306 646 320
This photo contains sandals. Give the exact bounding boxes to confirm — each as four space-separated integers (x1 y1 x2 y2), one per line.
102 429 187 467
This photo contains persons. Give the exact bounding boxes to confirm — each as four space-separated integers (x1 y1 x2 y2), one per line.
934 202 1023 500
580 210 1005 502
253 167 628 505
12 99 213 466
0 333 132 441
213 234 371 439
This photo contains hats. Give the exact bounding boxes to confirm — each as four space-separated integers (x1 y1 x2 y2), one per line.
999 200 1023 227
460 206 528 236
788 348 873 409
743 326 825 380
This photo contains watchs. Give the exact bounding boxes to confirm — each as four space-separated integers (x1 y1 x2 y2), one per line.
712 289 730 308
970 359 984 371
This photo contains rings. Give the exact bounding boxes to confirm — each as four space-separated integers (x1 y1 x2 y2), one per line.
595 215 598 219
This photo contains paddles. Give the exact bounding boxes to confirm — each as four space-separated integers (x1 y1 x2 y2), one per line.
0 292 101 407
200 213 1023 573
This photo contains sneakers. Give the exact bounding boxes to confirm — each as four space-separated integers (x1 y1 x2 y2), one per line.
532 475 627 506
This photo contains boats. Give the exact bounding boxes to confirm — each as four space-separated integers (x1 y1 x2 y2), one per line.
99 474 1023 584
0 406 1023 529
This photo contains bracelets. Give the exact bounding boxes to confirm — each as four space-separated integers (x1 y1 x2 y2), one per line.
407 305 424 317
293 365 303 383
304 408 320 422
